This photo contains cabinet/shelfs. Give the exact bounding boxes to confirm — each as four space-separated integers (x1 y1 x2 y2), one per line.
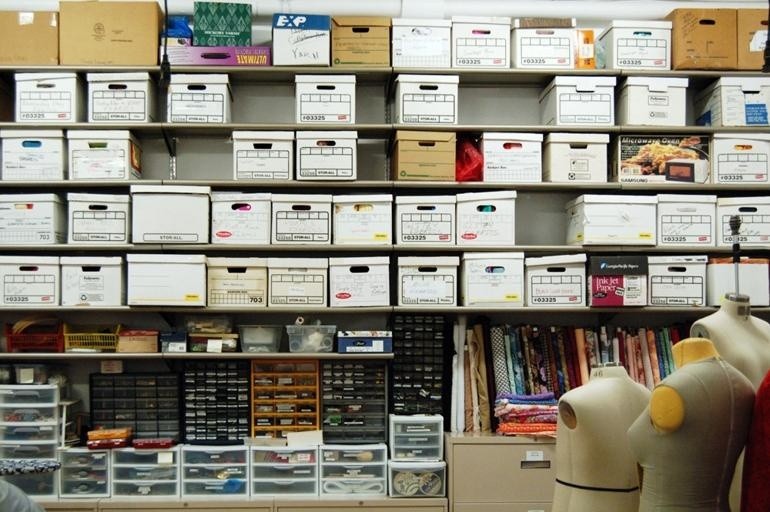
444 429 568 511
